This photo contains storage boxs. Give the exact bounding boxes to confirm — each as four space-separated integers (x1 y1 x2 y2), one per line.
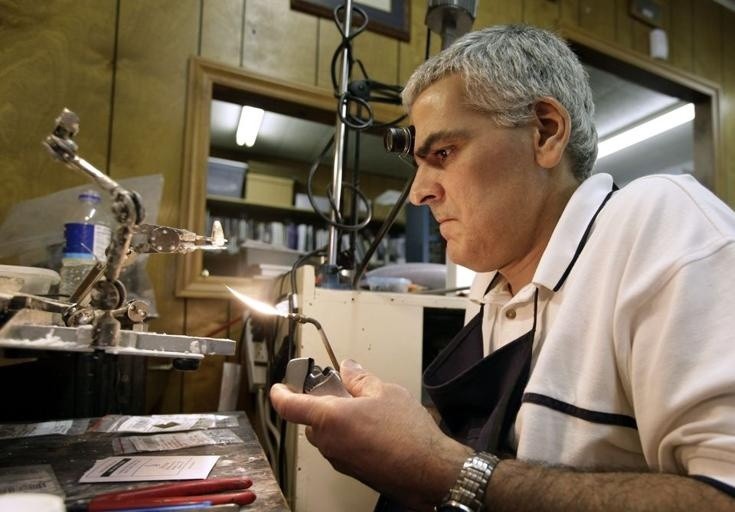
206 156 248 197
244 173 295 205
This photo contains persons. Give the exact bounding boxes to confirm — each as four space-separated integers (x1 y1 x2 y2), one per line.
268 25 735 511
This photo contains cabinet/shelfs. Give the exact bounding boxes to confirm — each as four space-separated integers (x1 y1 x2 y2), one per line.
204 193 406 262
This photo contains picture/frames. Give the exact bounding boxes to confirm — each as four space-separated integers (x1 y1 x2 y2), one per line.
290 0 412 43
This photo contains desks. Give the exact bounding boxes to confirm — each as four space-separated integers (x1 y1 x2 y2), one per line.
0 410 291 512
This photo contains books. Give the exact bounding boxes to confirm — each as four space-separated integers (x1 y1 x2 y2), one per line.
205 212 406 265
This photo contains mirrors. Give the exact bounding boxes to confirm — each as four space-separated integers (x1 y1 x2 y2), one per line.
174 55 456 300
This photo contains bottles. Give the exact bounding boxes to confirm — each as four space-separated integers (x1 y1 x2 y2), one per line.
61 191 114 300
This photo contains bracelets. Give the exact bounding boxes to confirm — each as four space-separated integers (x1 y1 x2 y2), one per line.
441 449 501 511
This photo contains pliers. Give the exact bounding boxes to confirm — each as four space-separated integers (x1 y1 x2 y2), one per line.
64 476 257 512
